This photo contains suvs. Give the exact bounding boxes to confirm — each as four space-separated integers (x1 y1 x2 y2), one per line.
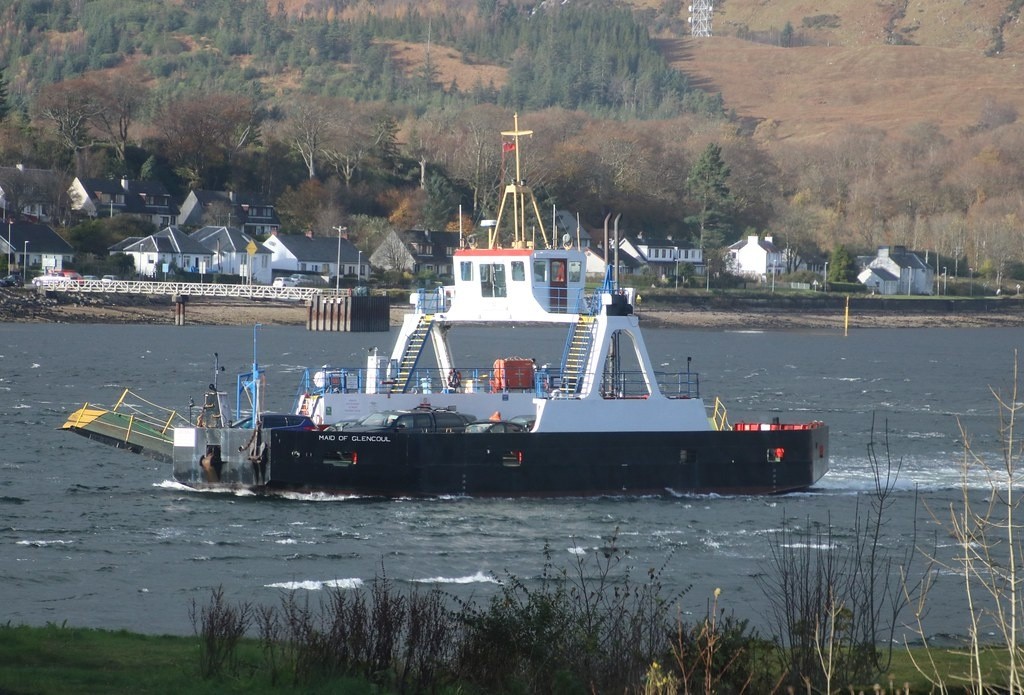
322 402 479 435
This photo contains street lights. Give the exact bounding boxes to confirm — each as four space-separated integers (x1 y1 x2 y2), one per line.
707 258 711 291
674 246 680 289
907 265 912 295
772 259 777 292
824 262 829 292
943 266 947 295
138 243 145 291
969 267 973 297
23 240 30 283
331 225 347 297
358 250 363 281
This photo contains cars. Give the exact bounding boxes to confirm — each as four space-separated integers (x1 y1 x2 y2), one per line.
461 411 529 434
0 269 120 289
231 412 318 431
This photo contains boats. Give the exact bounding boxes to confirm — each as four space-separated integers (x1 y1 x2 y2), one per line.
169 107 834 497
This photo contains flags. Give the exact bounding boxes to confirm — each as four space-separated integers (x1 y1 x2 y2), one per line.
503 143 515 152
